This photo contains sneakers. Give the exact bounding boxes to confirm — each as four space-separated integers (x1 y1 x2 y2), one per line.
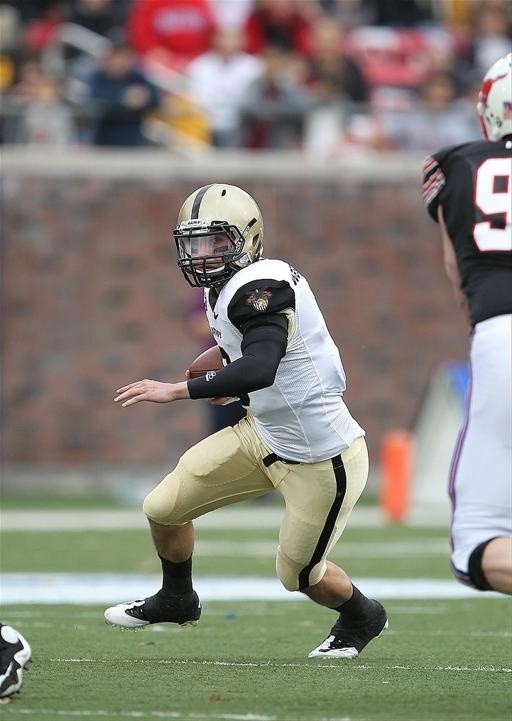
0 622 33 697
307 600 390 662
105 590 200 629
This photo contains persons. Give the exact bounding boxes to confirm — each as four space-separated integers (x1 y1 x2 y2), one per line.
101 182 387 659
1 1 512 163
424 50 512 591
180 287 274 500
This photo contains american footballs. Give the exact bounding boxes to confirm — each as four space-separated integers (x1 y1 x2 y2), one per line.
186 345 226 380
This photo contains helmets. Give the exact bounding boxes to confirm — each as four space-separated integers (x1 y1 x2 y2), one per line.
475 51 510 143
173 184 263 288
